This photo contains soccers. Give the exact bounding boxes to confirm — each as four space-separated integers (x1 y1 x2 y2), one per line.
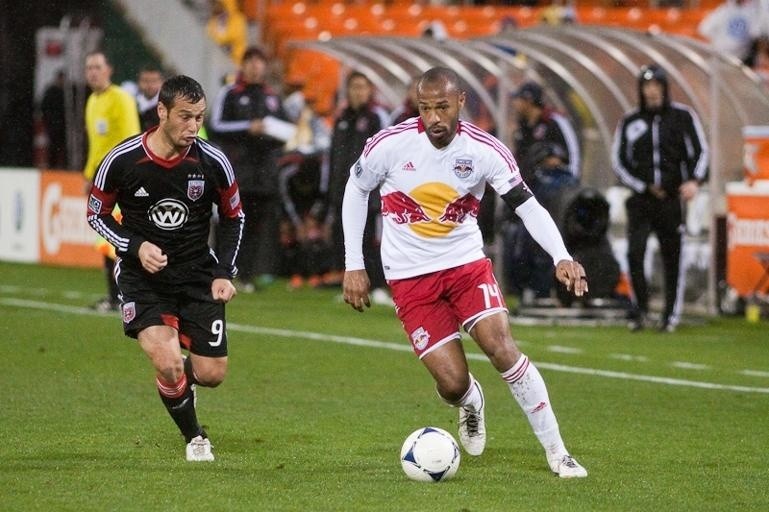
399 424 461 482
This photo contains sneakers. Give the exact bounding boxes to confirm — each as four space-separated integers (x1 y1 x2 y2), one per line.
456 379 487 457
88 298 120 312
184 432 214 462
181 352 197 409
545 450 589 480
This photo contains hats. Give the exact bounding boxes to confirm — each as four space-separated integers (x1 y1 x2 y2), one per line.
508 81 543 104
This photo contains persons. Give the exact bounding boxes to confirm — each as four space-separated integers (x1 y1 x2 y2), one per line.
609 63 710 333
279 150 330 289
40 69 77 169
390 76 420 125
209 44 292 293
133 64 166 131
501 80 580 272
83 50 141 312
86 76 247 460
342 67 590 478
327 71 389 286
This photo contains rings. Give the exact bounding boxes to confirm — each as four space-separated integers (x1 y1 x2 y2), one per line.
579 275 588 282
